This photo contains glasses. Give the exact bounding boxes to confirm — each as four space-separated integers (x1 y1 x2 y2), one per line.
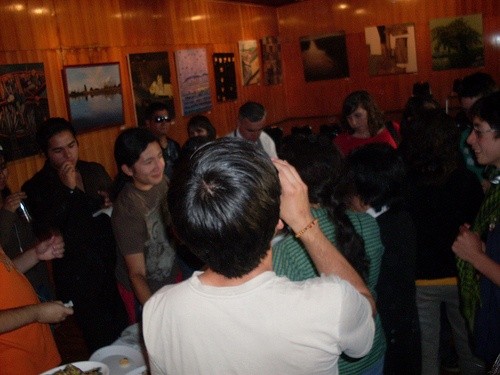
472 126 500 139
149 115 171 123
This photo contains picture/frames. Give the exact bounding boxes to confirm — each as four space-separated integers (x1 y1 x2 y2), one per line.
62 61 125 136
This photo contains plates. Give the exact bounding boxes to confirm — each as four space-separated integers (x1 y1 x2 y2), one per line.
88 345 148 375
40 361 109 375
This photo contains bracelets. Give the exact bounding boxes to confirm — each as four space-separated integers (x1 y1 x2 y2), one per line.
296 219 317 238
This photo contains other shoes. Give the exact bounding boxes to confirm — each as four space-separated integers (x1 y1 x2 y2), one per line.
445 360 460 371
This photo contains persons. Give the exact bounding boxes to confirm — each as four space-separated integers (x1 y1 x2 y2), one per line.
272 139 387 375
226 99 278 160
20 117 131 355
111 126 187 322
144 101 179 178
141 136 374 375
186 115 217 138
0 150 58 303
0 233 73 375
319 72 500 375
